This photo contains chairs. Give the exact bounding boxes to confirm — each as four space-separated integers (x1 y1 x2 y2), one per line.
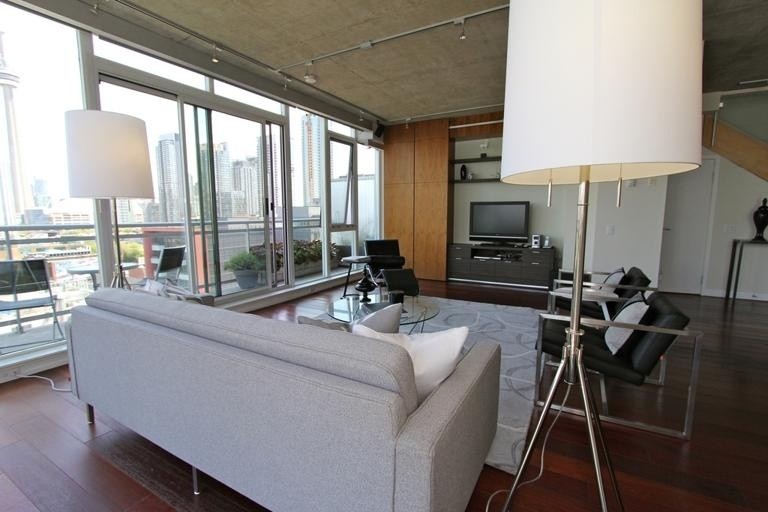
340 239 421 305
0 259 64 345
130 246 188 289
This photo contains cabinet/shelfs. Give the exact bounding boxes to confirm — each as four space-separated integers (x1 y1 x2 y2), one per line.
448 244 557 292
383 119 455 283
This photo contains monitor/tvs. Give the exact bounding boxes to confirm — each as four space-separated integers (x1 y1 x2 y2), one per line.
468 201 530 247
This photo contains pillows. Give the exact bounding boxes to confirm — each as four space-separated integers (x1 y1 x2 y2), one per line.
347 322 477 407
352 304 403 336
295 316 351 336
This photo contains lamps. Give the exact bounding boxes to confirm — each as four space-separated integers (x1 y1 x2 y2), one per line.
64 110 157 291
500 0 704 512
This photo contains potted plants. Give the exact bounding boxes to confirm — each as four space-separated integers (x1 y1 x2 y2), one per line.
222 251 267 290
248 240 339 286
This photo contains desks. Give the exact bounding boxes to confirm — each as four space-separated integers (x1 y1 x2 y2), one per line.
63 262 142 293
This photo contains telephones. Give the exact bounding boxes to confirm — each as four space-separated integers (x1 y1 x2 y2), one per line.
543 235 553 249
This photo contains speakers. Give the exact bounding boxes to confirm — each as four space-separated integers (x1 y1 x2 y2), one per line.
532 235 540 248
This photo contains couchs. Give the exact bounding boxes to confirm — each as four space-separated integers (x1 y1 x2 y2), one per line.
65 285 504 512
548 266 652 320
533 289 704 442
